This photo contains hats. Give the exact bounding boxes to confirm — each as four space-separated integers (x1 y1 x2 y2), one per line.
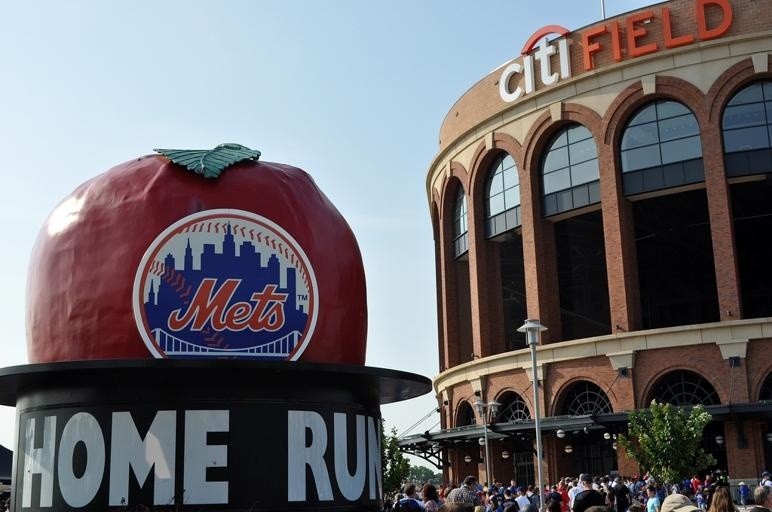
635 481 646 489
762 471 770 477
581 474 594 482
446 487 474 507
661 493 705 512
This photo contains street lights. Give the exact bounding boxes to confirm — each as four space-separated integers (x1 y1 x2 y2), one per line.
471 397 501 485
515 317 549 512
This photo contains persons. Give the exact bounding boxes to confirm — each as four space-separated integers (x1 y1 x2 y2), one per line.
0 498 10 512
383 469 771 511
